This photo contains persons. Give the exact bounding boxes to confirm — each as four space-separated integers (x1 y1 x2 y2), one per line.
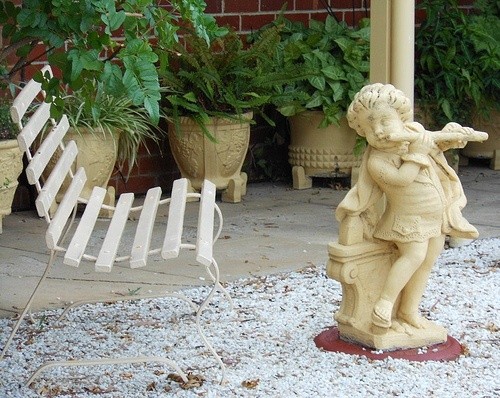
336 84 479 329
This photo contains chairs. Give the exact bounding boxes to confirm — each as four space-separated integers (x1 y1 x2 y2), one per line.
0 64 234 387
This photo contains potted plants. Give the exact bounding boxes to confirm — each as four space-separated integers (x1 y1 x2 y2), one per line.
0 95 31 234
108 2 324 202
244 0 372 189
0 0 229 221
414 0 500 172
